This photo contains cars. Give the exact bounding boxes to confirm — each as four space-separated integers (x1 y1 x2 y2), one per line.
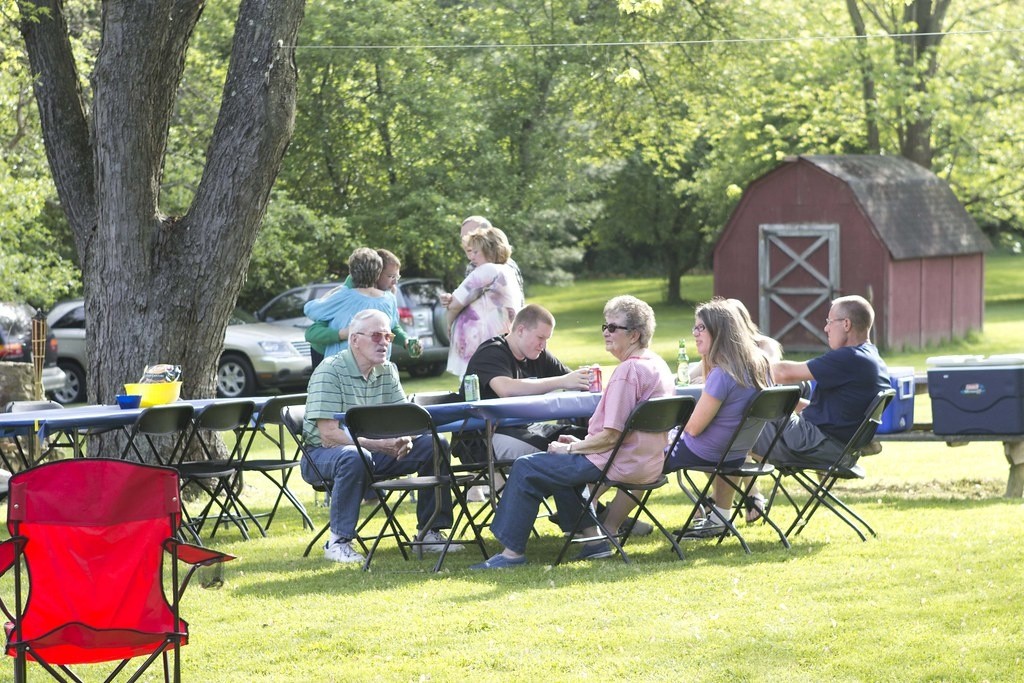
39 294 313 404
0 289 68 403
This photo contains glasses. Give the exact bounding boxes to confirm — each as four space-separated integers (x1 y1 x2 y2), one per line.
826 318 845 325
602 324 628 333
382 271 401 280
692 324 707 332
356 331 396 342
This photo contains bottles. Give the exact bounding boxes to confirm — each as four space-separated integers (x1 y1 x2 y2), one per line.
677 337 690 386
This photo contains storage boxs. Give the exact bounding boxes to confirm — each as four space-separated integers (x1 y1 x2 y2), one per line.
925 356 1024 435
874 367 916 434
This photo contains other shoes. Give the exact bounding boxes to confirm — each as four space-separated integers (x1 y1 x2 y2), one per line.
672 528 693 537
745 494 765 522
619 517 653 535
696 496 715 517
683 517 736 539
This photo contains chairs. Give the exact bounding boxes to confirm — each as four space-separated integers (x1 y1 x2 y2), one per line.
3 384 900 573
0 460 236 683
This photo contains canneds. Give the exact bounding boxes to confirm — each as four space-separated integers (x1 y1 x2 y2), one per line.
408 338 420 358
578 365 603 394
463 373 481 401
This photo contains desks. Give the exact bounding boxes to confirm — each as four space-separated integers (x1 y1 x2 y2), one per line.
0 393 277 530
335 380 815 514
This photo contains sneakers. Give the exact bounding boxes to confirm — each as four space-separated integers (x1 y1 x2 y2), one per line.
570 541 613 558
412 531 465 552
468 553 527 570
324 537 364 563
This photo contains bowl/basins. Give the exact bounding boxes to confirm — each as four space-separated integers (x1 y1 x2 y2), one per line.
116 395 142 409
124 381 183 406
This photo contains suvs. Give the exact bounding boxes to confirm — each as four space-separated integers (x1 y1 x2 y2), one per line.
249 272 453 381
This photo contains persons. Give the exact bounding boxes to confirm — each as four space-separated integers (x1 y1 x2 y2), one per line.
673 298 783 524
460 294 674 571
548 302 777 546
448 302 659 537
437 213 529 378
670 294 891 541
301 308 467 566
301 246 422 379
